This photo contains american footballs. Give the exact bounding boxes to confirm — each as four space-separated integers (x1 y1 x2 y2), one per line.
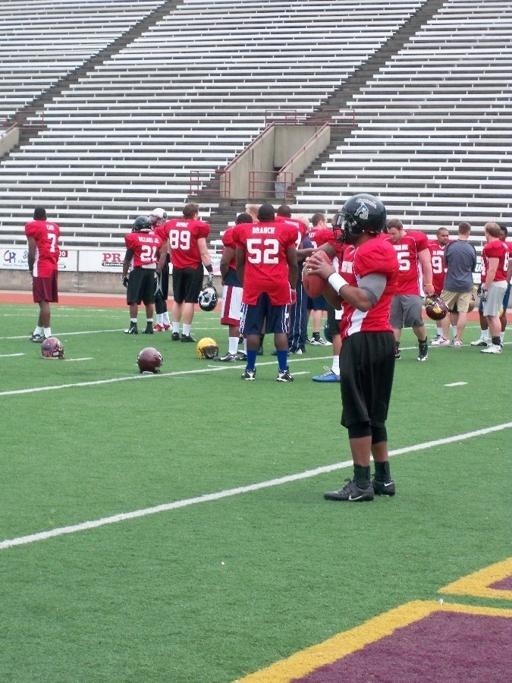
300 248 332 300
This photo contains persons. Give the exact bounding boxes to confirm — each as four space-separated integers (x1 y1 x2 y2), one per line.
306 212 335 347
255 205 308 357
121 214 164 335
301 193 400 503
148 207 173 333
231 202 299 384
383 219 436 362
470 225 512 348
480 221 509 354
428 221 477 347
212 213 254 363
311 243 355 383
154 203 214 343
427 227 464 346
287 236 313 356
24 206 61 345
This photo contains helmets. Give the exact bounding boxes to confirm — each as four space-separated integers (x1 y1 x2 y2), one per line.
137 346 163 374
133 216 150 233
40 337 65 360
426 294 448 320
198 285 218 312
332 193 386 244
151 208 168 223
196 338 218 361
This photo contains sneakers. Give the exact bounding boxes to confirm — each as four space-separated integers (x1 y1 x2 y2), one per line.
394 350 401 358
32 331 40 342
431 336 450 346
181 334 195 342
153 324 165 332
35 336 52 343
417 344 429 361
142 328 153 334
452 336 462 346
163 321 172 331
318 338 332 346
236 353 248 361
480 343 503 354
371 479 395 496
124 328 138 334
471 339 489 346
324 479 374 502
306 338 321 346
276 370 296 383
241 369 256 381
311 369 341 382
213 352 237 362
172 332 180 340
